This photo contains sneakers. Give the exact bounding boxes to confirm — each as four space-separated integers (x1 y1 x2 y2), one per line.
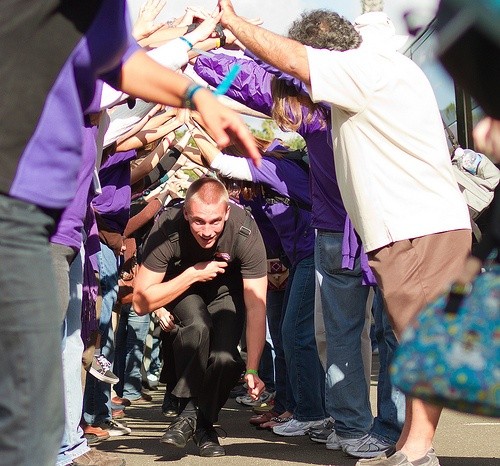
192 425 227 457
160 415 197 449
86 353 119 384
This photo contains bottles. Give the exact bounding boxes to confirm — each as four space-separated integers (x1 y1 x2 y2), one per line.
454 146 481 176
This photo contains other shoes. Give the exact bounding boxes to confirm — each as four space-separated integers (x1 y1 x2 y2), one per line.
339 433 397 458
229 376 276 414
147 374 159 382
65 450 126 466
308 420 334 443
143 381 158 391
117 420 128 426
326 429 358 450
92 420 132 436
81 433 99 443
82 425 111 441
273 419 324 436
111 401 125 411
356 444 439 466
249 408 293 430
130 393 152 403
162 393 178 417
112 396 131 407
112 409 124 419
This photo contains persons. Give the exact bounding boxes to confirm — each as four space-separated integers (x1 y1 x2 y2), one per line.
0 0 500 466
131 177 269 456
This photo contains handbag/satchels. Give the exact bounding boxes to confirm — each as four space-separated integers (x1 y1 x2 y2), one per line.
450 147 500 221
387 224 500 418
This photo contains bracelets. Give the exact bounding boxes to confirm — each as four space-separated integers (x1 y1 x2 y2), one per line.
165 136 172 147
184 83 203 110
214 34 227 48
154 197 164 207
244 369 259 377
180 36 194 48
